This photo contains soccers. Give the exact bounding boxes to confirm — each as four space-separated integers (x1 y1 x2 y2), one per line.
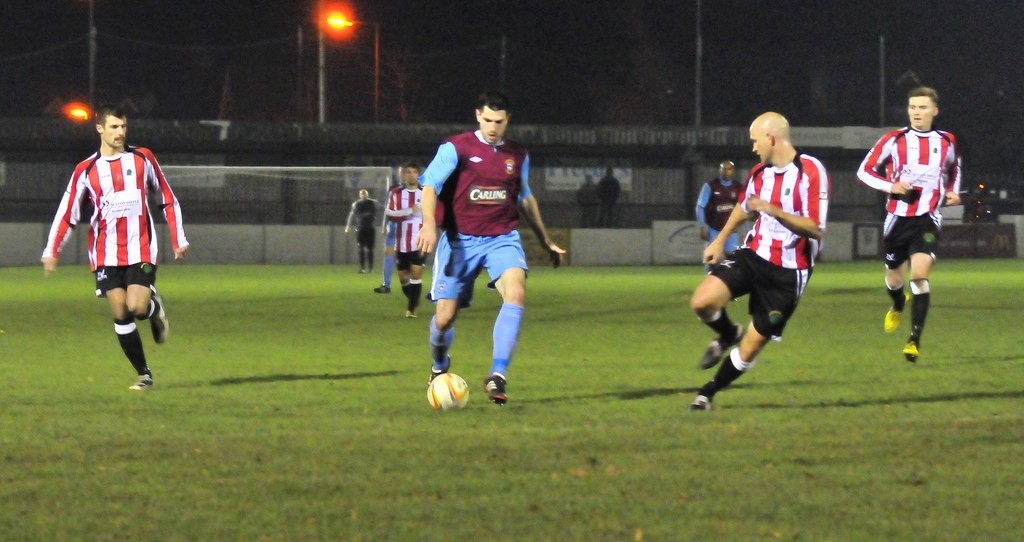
427 372 469 410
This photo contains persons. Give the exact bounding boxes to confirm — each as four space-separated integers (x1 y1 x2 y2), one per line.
415 91 564 405
580 175 598 228
694 160 742 274
373 163 406 294
690 112 832 409
39 102 190 391
856 86 964 362
596 168 619 228
384 162 429 317
344 188 383 274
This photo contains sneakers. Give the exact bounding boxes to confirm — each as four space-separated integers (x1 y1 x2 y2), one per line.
483 375 508 405
428 355 450 385
884 290 909 333
903 342 919 363
700 324 742 369
130 373 153 390
691 396 712 411
150 295 169 343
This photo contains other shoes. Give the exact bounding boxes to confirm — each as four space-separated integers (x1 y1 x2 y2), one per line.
359 269 365 273
405 310 416 318
373 285 390 293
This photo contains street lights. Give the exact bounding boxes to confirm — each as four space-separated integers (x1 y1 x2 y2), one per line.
327 16 380 124
